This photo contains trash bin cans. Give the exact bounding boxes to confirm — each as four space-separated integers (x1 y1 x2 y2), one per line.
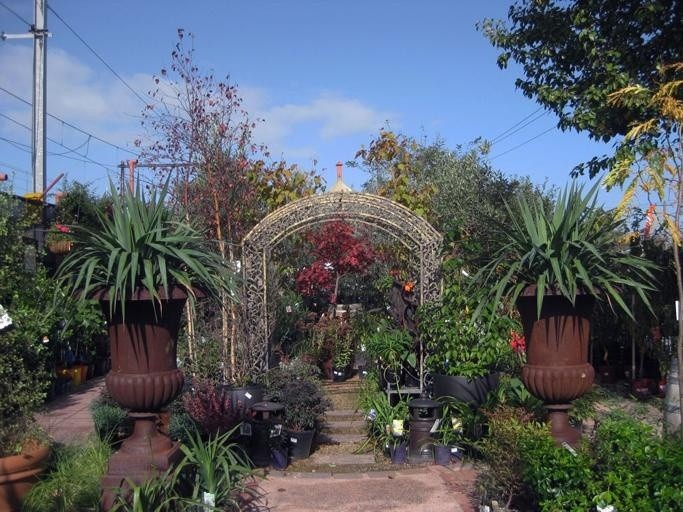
251 401 285 468
406 398 442 464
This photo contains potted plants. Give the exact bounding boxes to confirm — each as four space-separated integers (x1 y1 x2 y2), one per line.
3 171 677 508
